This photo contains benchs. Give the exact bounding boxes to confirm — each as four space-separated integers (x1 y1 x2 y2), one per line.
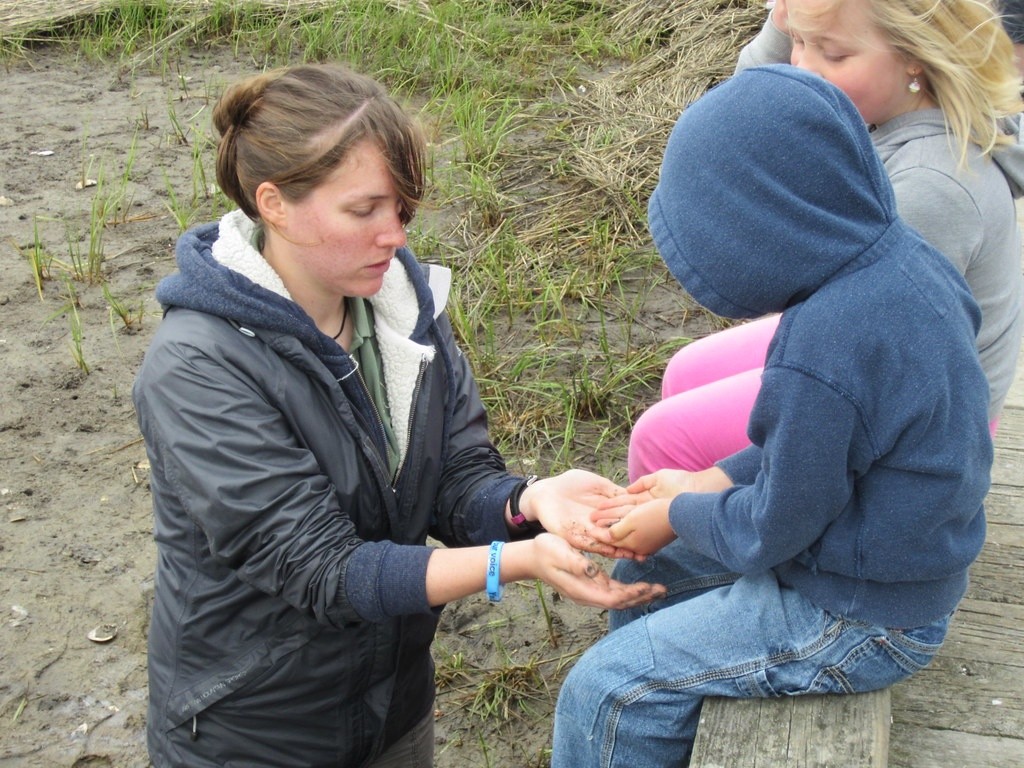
691 692 893 767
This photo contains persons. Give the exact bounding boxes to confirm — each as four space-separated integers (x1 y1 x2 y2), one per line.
627 0 1024 487
132 64 666 768
554 65 989 768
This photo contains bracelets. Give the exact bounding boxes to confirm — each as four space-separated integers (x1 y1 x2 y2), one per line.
486 541 505 602
510 475 541 531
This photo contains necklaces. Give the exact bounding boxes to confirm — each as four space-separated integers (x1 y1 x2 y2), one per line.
332 297 349 340
